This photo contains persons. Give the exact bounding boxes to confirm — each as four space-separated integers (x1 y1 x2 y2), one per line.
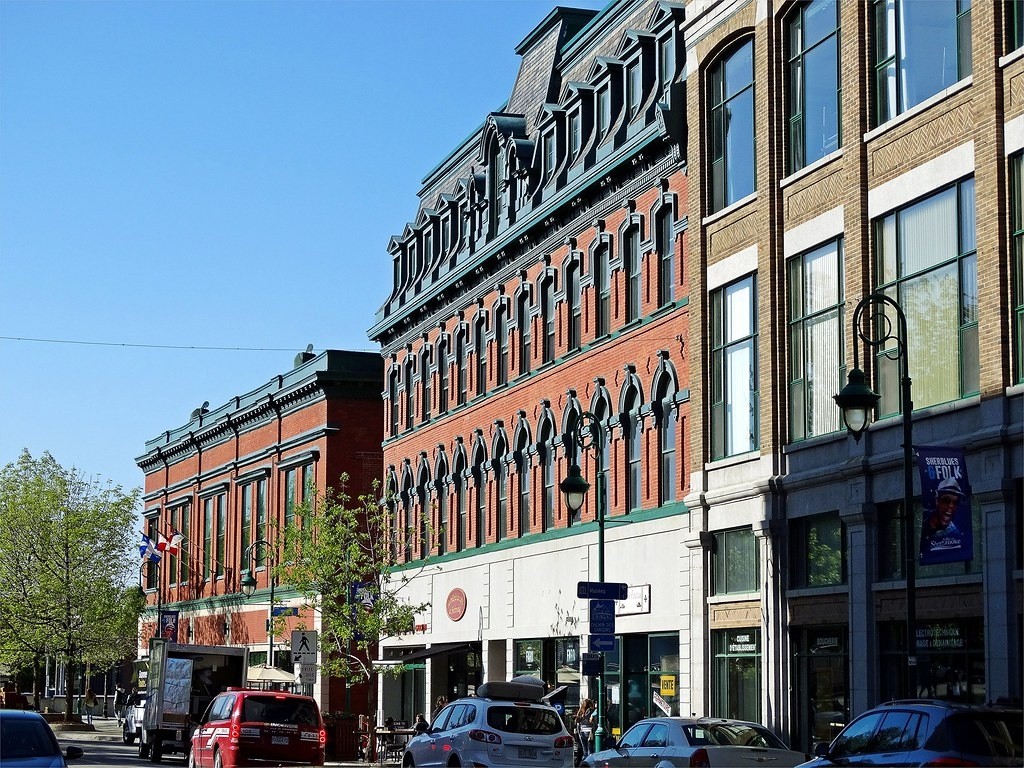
127 687 137 709
410 713 428 729
577 698 612 760
918 669 938 699
82 688 96 725
921 477 964 542
431 695 449 722
113 682 126 728
384 717 402 758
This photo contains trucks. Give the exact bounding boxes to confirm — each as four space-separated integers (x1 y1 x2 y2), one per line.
139 643 250 761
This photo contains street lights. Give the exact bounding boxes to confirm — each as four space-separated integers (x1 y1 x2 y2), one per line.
835 292 966 702
240 541 274 666
136 561 161 637
71 637 83 717
560 412 633 754
321 512 351 713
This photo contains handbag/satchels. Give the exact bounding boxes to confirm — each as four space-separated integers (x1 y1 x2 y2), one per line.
94 696 98 706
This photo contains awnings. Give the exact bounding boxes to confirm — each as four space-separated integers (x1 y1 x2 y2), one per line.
132 656 149 662
403 643 472 665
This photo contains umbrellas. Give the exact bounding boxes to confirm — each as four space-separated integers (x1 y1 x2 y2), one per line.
247 662 298 691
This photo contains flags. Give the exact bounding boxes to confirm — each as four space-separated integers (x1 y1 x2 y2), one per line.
153 532 168 553
139 534 163 563
165 526 186 556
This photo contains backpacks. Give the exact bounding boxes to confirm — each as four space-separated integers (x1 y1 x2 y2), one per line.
116 689 127 705
129 693 141 705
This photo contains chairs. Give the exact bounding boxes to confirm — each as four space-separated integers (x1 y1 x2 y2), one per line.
374 727 426 764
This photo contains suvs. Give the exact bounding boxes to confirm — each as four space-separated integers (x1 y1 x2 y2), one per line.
188 686 327 768
401 696 575 768
793 699 1024 768
123 691 146 746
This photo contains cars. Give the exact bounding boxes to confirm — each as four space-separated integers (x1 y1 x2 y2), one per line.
579 717 812 768
0 709 83 768
811 700 845 741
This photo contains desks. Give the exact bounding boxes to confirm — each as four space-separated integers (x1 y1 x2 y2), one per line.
376 730 417 768
